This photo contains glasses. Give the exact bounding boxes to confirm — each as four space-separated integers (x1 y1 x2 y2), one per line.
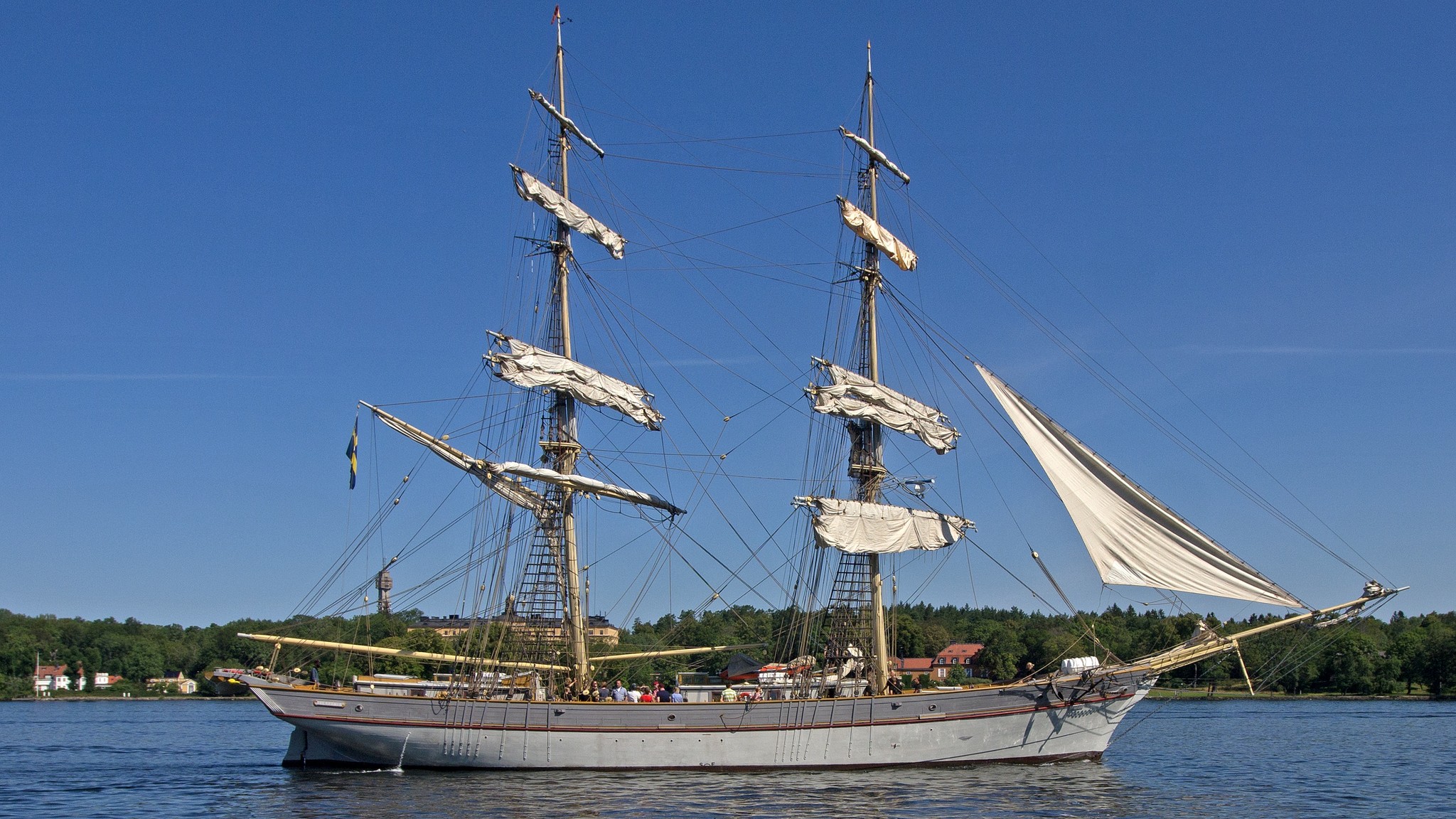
594 683 597 684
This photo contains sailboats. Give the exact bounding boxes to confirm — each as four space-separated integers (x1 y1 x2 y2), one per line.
242 8 1412 772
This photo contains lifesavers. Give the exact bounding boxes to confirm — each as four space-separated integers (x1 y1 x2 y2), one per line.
738 692 753 700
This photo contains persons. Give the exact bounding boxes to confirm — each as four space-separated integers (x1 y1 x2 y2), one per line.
720 682 737 703
1012 662 1037 684
598 682 610 702
310 659 322 689
560 677 577 703
640 687 655 703
583 672 593 691
863 681 876 696
656 684 672 703
627 683 641 703
881 670 903 695
912 677 922 694
609 680 627 702
671 687 684 703
590 681 601 702
750 687 763 701
333 675 340 692
639 681 660 703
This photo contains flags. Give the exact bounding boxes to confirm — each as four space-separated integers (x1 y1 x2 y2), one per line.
346 413 358 489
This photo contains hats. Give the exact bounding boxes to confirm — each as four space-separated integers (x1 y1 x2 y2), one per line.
654 682 659 684
631 683 638 689
657 683 664 687
